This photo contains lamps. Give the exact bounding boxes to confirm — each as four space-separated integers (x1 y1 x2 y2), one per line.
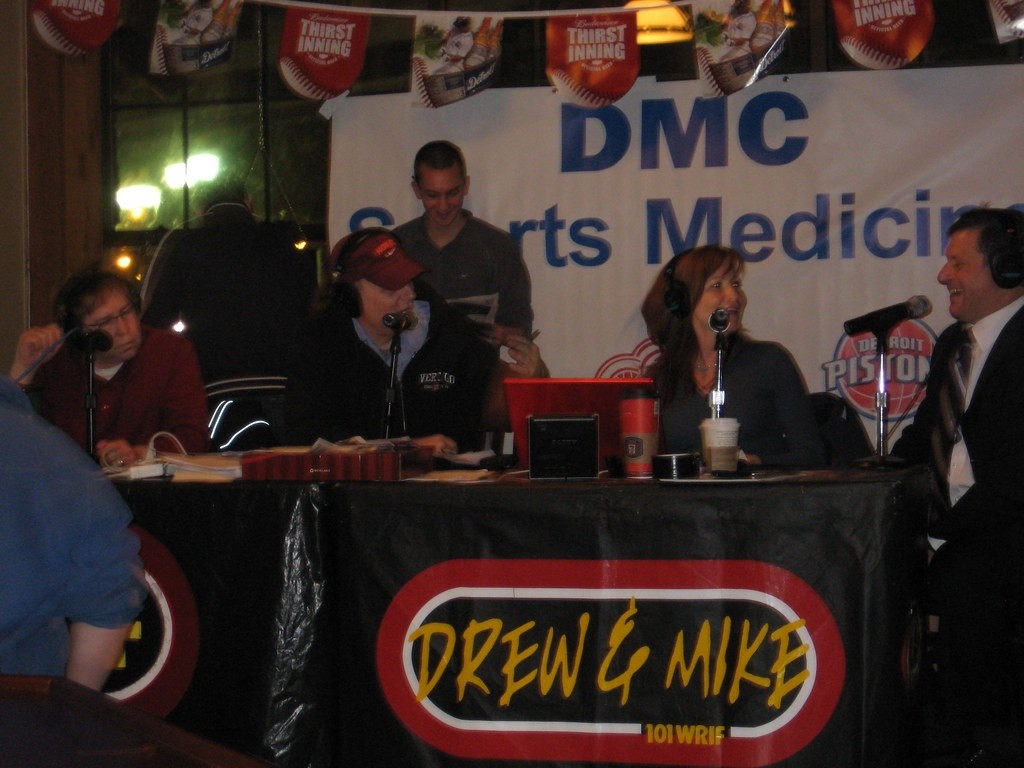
623 0 693 45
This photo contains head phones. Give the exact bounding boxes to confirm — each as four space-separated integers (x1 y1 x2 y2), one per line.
331 225 401 316
53 273 144 331
992 210 1024 290
665 248 695 317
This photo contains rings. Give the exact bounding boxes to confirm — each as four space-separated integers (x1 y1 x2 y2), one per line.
119 458 126 466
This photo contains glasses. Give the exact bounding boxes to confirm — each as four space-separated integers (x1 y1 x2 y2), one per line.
80 295 140 333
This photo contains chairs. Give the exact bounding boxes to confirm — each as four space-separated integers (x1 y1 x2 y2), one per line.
811 392 874 465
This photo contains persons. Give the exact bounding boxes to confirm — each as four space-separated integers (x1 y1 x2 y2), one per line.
887 207 1024 768
1 369 148 693
636 244 828 470
140 167 320 453
9 266 212 465
388 140 535 350
300 226 552 470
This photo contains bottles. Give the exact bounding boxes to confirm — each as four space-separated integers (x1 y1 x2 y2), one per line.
749 0 786 51
463 15 503 70
199 0 243 44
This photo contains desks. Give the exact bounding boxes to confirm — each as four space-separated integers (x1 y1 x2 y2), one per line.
99 458 926 767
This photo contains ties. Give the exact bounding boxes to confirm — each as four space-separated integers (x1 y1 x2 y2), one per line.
926 325 978 522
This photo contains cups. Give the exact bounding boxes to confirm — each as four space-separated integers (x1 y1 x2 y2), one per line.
616 386 662 478
650 452 700 479
699 418 741 474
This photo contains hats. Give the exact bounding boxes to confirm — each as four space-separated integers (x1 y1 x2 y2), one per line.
329 230 427 292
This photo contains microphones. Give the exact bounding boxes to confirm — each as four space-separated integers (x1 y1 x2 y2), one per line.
382 309 419 331
73 331 113 352
708 309 730 332
844 294 934 337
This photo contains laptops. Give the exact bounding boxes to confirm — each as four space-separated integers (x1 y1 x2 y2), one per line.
503 377 654 474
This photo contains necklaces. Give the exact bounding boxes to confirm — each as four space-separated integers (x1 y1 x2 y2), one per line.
694 363 719 367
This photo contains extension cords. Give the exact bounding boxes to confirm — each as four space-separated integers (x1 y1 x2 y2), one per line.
127 462 165 479
